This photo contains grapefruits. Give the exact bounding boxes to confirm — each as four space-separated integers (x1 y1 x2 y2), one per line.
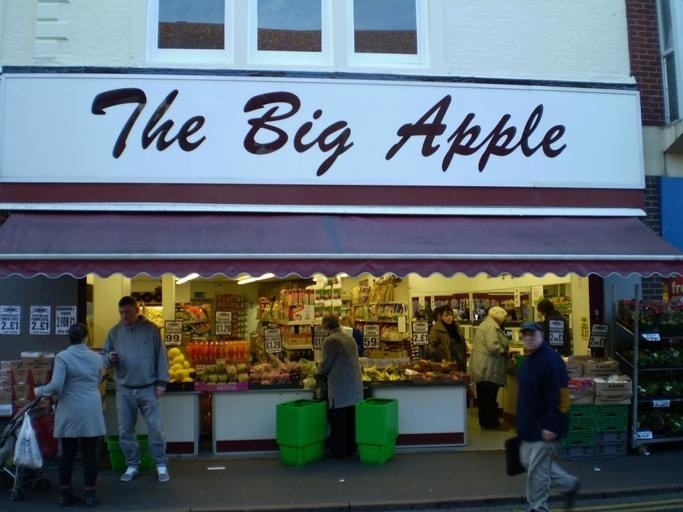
167 347 195 384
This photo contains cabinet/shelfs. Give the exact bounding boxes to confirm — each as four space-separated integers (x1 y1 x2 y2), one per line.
609 283 683 456
255 300 402 369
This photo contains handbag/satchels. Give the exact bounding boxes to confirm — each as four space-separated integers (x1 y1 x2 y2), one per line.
33 405 57 458
505 436 527 476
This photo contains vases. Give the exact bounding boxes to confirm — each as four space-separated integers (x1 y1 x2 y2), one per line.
624 317 680 336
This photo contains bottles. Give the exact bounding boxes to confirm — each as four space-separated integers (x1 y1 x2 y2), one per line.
185 340 250 364
280 288 314 305
281 325 311 335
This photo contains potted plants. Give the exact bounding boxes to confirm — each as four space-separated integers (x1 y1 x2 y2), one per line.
628 348 683 435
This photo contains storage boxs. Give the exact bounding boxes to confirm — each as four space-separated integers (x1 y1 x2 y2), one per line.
564 355 633 405
0 356 54 416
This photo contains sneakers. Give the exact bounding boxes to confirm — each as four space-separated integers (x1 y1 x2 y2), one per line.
564 478 581 509
120 465 139 482
156 466 170 483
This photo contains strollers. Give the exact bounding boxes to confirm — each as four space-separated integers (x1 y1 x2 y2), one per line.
0 393 53 502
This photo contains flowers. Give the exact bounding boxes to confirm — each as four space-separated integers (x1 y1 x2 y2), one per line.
619 297 683 328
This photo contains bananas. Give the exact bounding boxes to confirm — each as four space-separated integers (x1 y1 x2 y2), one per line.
361 364 405 383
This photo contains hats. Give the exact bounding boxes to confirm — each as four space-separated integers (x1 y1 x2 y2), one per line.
519 321 546 333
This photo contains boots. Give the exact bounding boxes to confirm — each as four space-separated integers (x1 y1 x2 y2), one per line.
85 486 97 508
60 485 78 506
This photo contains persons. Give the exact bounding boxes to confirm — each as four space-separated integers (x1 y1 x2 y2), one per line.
469 306 515 431
316 312 363 459
337 313 365 359
33 321 107 508
536 299 571 357
409 300 488 409
513 320 581 512
99 295 171 483
